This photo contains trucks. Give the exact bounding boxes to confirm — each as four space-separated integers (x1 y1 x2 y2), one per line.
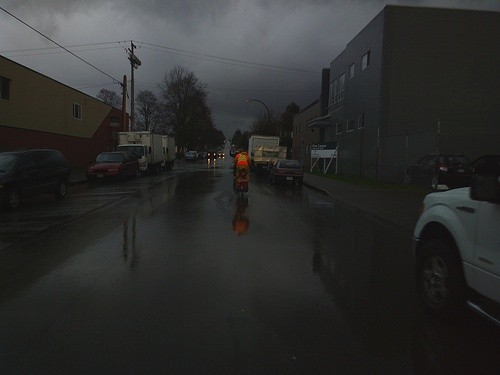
118 131 177 176
248 135 279 171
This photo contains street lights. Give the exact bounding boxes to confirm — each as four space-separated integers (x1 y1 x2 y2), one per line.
248 98 271 119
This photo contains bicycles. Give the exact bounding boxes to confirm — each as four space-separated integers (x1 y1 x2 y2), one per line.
232 166 249 199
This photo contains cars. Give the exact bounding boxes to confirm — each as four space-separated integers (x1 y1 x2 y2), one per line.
410 153 500 326
406 151 472 192
264 159 304 185
0 147 70 211
185 150 226 159
87 152 133 183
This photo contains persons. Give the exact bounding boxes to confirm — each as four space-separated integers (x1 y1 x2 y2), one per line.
231 207 250 237
234 146 250 177
